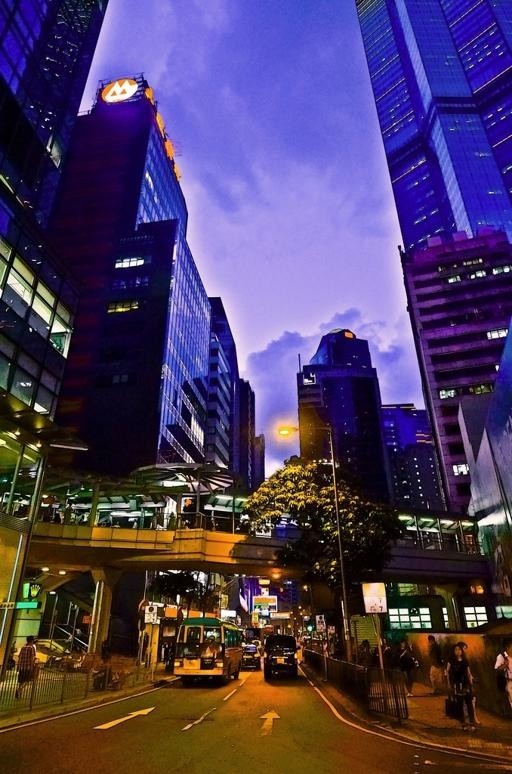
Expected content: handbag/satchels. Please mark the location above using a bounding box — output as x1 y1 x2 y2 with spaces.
445 699 458 720
494 653 508 690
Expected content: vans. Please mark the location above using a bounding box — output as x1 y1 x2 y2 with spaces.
264 636 298 681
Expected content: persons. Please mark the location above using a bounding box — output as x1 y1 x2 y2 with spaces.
0 494 113 528
376 633 420 698
156 642 175 663
16 635 36 699
101 640 112 663
67 630 77 643
428 635 481 734
494 636 512 708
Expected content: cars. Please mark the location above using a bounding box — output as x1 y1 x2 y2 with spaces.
240 645 261 672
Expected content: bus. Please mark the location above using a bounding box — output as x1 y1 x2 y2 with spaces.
173 617 241 683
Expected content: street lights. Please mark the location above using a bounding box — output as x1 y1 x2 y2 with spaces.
29 437 90 522
277 424 353 662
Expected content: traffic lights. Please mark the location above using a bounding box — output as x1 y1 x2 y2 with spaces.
306 621 314 632
